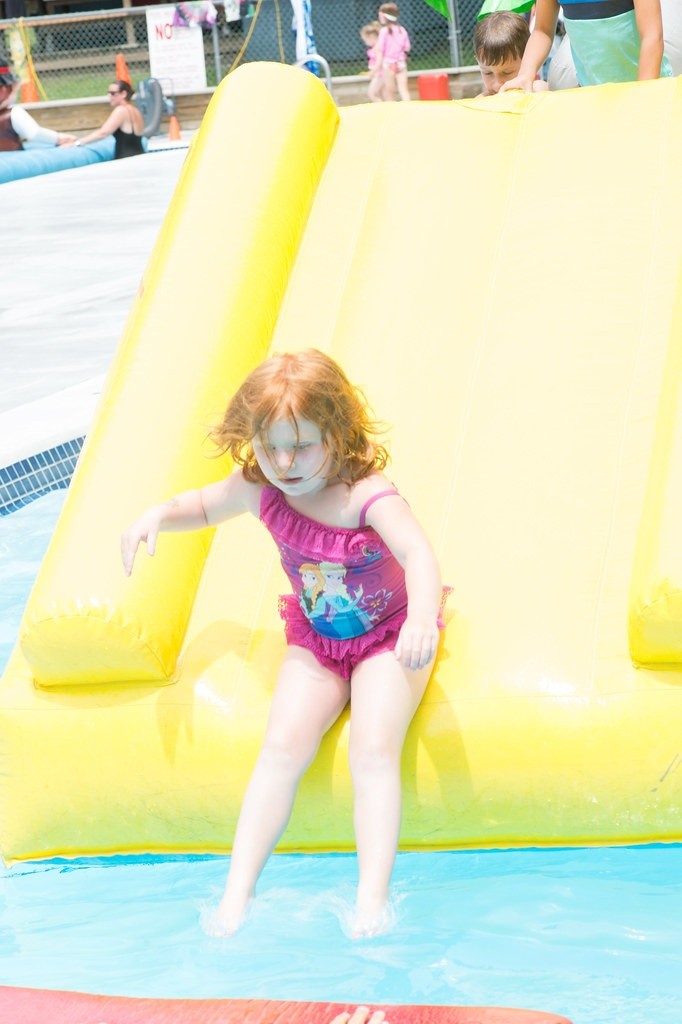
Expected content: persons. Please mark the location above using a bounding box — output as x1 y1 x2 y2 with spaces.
529 2 580 91
0 73 76 151
360 4 410 102
60 80 144 159
474 9 548 98
500 0 673 94
120 351 443 935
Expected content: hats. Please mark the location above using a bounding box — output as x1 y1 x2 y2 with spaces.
0 55 20 87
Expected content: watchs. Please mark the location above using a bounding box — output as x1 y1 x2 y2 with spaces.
74 140 80 146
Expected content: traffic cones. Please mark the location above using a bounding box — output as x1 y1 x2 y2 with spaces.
19 59 41 104
115 52 132 90
168 116 181 142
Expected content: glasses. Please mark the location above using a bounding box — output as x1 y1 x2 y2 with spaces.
107 90 119 96
